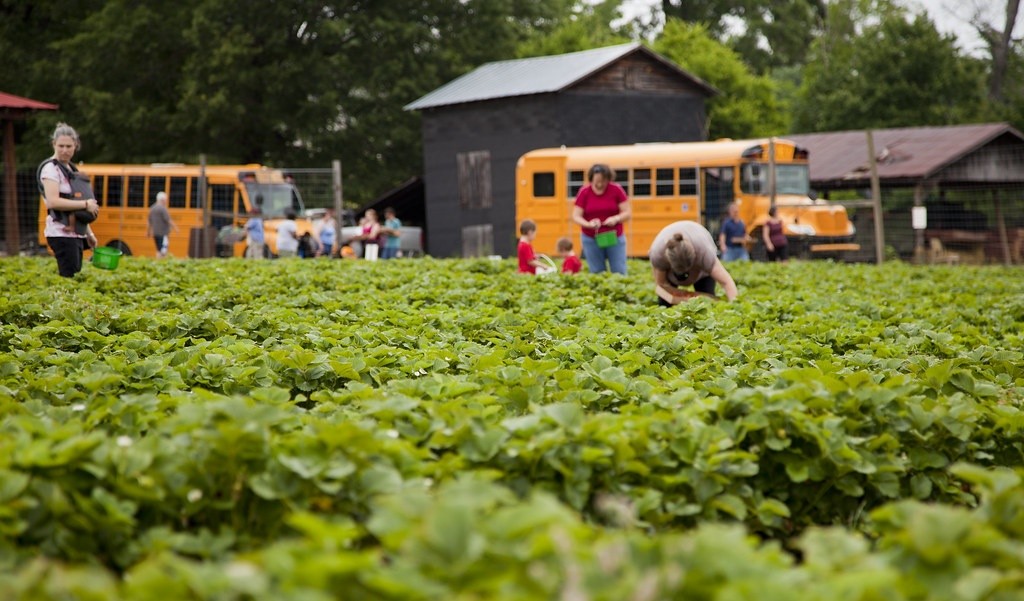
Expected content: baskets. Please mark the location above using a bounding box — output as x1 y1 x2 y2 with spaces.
595 223 617 247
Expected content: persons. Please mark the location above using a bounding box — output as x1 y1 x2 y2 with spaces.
558 239 582 273
649 221 737 308
147 191 177 258
763 206 787 260
353 209 380 258
315 212 334 255
380 208 402 259
564 164 630 268
719 205 749 260
36 123 99 277
240 210 264 259
517 220 548 274
275 213 304 257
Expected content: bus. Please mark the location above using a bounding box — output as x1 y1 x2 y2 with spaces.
39 162 323 258
515 138 861 261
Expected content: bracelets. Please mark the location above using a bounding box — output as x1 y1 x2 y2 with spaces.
88 234 94 237
86 201 88 208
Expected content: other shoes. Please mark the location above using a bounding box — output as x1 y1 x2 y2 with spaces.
296 230 318 257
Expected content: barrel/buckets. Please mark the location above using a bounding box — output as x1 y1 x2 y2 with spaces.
92 246 123 270
596 224 617 248
